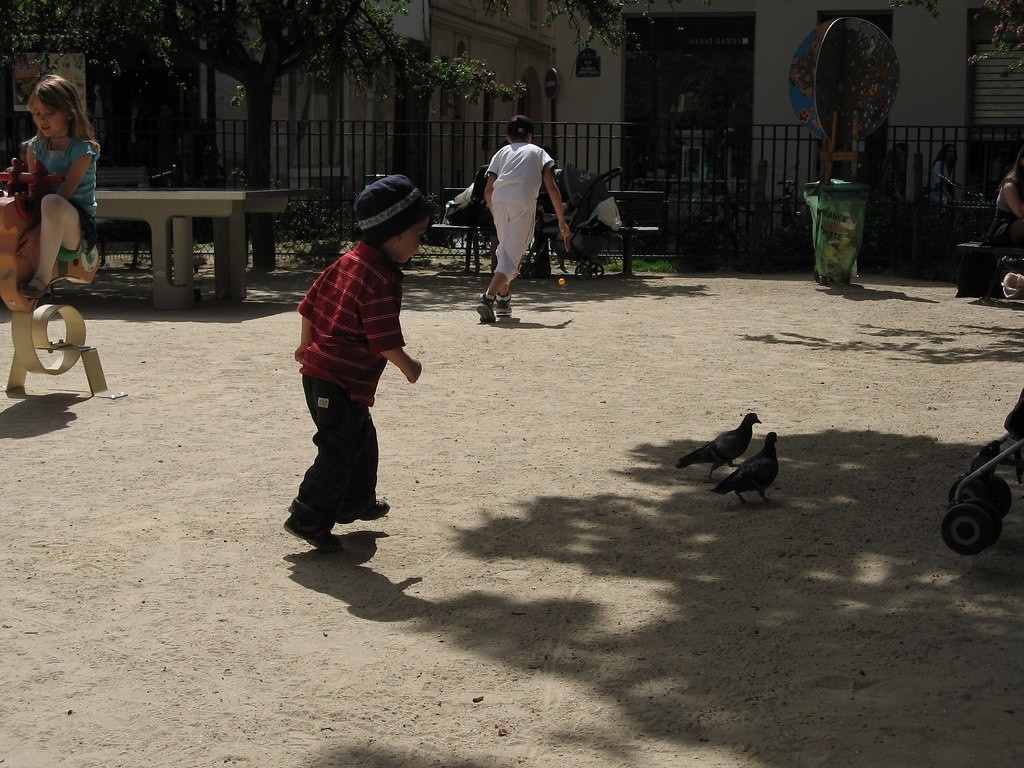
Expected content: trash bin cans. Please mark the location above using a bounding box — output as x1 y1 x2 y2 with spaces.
803 178 872 286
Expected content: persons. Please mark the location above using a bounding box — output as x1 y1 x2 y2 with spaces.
11 73 97 300
283 175 423 553
929 144 959 205
476 115 570 323
883 142 910 199
988 143 1024 248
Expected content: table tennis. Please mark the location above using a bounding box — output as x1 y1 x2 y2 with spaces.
558 278 565 286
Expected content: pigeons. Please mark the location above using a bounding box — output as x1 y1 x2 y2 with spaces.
675 413 762 482
710 431 780 508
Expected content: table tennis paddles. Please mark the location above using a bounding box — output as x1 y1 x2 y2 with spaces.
561 226 571 252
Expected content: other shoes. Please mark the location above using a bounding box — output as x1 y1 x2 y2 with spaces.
21 275 53 298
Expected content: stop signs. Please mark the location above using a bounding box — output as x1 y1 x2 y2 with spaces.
544 69 558 99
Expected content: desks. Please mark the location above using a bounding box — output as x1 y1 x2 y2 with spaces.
93 186 324 311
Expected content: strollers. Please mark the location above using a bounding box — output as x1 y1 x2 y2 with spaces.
941 384 1024 556
517 163 624 282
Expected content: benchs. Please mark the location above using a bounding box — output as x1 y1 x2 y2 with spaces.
98 167 154 272
956 203 1024 303
432 190 666 279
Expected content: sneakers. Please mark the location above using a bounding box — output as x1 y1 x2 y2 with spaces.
281 512 344 553
494 291 515 318
340 499 390 524
475 292 498 323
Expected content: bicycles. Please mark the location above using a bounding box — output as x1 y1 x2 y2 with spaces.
681 177 800 271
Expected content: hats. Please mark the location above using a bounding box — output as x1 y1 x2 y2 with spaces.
507 114 535 138
353 173 438 246
